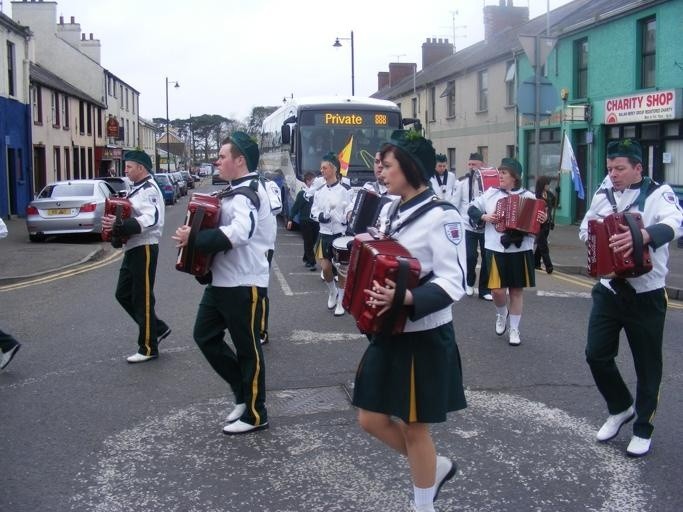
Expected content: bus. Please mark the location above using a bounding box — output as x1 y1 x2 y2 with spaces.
259 97 422 231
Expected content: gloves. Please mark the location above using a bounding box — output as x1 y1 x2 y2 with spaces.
321 210 331 220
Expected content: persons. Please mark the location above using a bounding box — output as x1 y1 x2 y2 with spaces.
532 174 558 275
430 153 458 201
579 141 683 456
468 157 548 347
344 149 398 233
0 217 23 372
309 153 354 317
303 155 353 282
258 168 278 345
353 131 465 512
102 151 171 363
287 172 320 271
272 169 289 227
169 132 270 435
451 153 494 301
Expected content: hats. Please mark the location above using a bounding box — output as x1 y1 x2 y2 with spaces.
435 153 447 162
384 127 436 183
500 156 523 176
468 151 484 162
122 149 153 171
321 151 339 168
228 128 260 171
605 137 643 164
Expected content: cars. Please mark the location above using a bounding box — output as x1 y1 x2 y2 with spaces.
153 173 180 205
211 170 230 185
100 177 136 194
198 167 207 177
178 171 196 189
166 171 187 196
191 172 201 181
25 179 126 240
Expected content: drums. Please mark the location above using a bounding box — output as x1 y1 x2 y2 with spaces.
470 166 500 229
330 234 356 266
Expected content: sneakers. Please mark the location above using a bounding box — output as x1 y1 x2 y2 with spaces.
320 269 324 279
625 435 652 458
303 259 308 266
547 266 553 276
482 293 493 301
535 265 541 269
1 342 21 368
433 455 457 503
157 327 171 345
326 287 340 310
126 351 160 366
334 298 346 316
223 402 248 423
223 418 270 436
333 274 340 282
257 330 268 344
595 405 636 442
509 329 521 346
310 263 316 271
495 309 510 336
465 284 474 296
